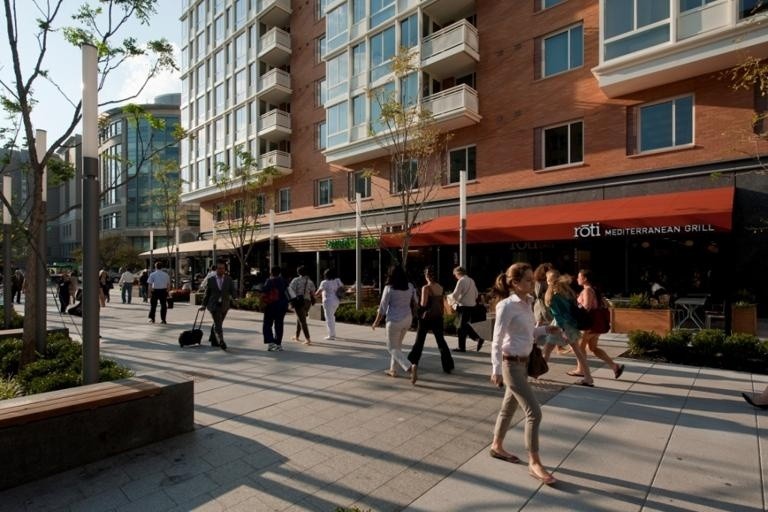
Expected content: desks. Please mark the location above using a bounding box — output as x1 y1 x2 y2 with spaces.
673 292 711 331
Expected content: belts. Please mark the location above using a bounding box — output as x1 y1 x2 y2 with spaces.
503 356 527 361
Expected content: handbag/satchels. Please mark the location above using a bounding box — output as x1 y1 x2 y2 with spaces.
410 291 419 329
469 304 486 324
336 279 345 299
528 343 549 379
290 295 305 308
571 308 594 330
590 306 610 334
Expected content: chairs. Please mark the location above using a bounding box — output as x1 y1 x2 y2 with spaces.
704 300 726 329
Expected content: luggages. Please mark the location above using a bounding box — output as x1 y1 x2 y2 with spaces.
179 307 205 347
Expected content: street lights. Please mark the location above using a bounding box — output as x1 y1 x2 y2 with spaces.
213 226 217 269
150 230 153 274
36 129 46 357
269 209 275 276
2 173 12 331
459 169 467 275
81 43 100 384
175 225 179 289
356 192 361 311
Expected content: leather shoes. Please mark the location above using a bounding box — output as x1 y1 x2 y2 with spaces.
452 348 466 352
490 447 520 465
476 338 484 351
528 463 557 485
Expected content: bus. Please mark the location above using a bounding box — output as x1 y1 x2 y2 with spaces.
46 257 80 282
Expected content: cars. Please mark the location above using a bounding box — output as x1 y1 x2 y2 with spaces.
77 264 175 284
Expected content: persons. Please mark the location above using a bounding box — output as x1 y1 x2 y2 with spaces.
198 263 239 350
288 264 315 344
315 267 345 340
373 261 419 384
447 264 485 353
741 384 767 411
408 264 455 375
475 263 626 389
10 259 176 324
489 262 557 484
261 266 290 352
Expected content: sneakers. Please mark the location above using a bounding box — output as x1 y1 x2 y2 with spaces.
410 364 417 384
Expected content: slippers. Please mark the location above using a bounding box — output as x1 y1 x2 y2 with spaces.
615 364 624 378
567 371 584 377
574 380 594 386
